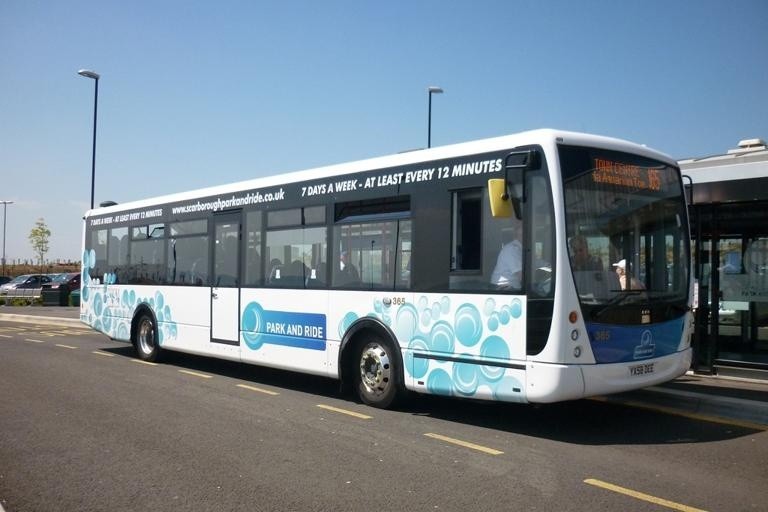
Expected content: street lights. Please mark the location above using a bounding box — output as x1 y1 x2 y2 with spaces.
427 86 444 148
78 69 100 210
0 200 13 276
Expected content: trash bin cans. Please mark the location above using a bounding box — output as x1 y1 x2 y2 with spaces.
42 283 69 305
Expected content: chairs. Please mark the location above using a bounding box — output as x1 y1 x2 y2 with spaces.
106 231 381 288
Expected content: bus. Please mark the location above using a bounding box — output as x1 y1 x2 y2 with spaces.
80 128 694 410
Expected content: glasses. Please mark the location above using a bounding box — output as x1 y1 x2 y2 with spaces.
573 247 585 253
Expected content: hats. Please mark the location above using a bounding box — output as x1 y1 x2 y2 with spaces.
612 259 632 272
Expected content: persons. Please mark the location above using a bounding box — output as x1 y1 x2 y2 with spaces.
489 220 537 290
221 235 237 275
340 251 359 280
612 259 647 291
569 236 602 274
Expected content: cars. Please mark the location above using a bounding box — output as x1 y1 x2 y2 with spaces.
0 272 80 296
